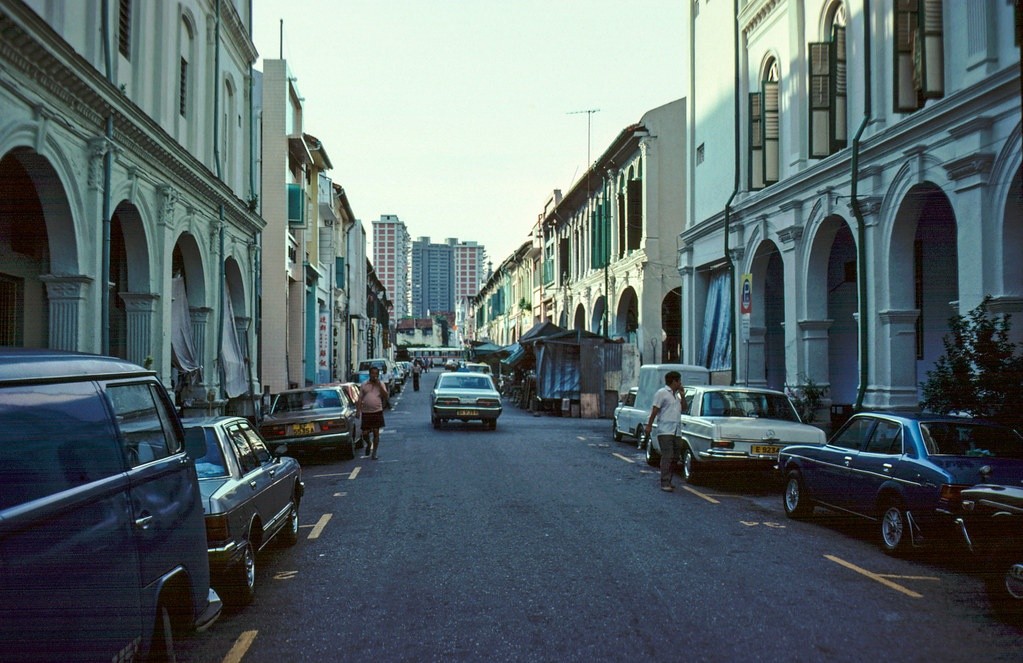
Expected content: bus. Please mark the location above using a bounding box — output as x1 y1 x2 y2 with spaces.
405 347 472 369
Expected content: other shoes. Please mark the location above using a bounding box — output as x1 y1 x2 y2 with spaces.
366 442 372 455
372 453 378 459
661 483 676 491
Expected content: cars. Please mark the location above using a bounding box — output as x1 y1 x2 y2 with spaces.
949 481 1023 626
430 356 495 378
771 407 1023 563
256 358 414 462
116 416 305 602
646 384 828 486
430 371 502 430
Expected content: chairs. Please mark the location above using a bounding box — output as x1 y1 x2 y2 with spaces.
477 378 488 388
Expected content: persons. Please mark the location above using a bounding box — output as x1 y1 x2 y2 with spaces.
356 367 389 461
412 361 420 392
450 359 458 366
408 357 429 377
645 371 688 491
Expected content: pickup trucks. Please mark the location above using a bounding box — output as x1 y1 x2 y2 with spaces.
614 386 654 453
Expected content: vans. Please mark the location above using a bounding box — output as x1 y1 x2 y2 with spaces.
0 349 224 663
